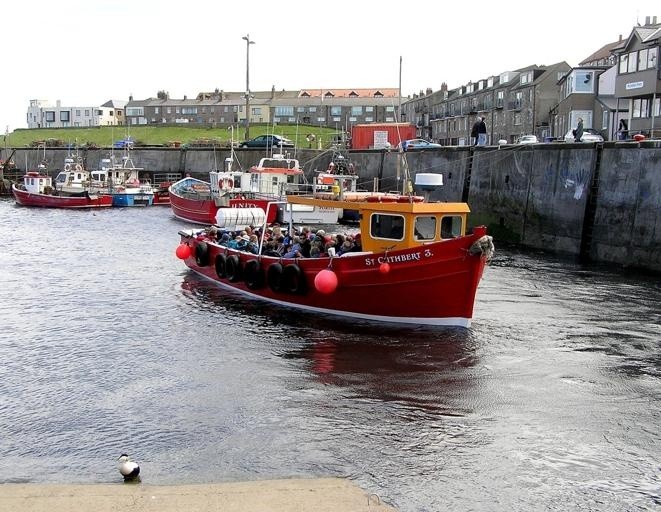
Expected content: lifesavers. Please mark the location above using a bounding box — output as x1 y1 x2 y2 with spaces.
75 164 82 172
64 163 71 171
306 132 315 143
26 171 38 176
367 195 423 203
218 176 233 191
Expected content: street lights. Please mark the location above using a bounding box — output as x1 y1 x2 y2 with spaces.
242 32 256 140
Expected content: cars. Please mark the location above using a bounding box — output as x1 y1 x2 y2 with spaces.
564 128 605 143
400 138 442 149
515 135 539 144
239 134 295 148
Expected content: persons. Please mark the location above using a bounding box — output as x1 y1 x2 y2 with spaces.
573 117 585 141
469 116 487 146
617 119 628 141
196 224 361 258
389 217 403 238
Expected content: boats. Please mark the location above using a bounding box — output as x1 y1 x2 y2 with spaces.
244 149 424 226
54 135 156 208
176 173 496 331
166 157 278 227
12 154 115 209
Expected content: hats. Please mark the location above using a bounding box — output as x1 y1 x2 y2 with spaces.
313 230 355 251
